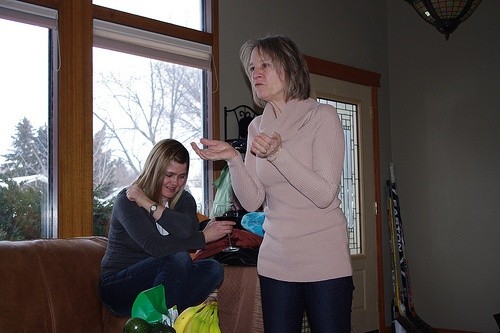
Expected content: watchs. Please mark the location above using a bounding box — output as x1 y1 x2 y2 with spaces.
150 203 159 216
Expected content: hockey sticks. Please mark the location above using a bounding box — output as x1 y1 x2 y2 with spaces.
384 162 435 333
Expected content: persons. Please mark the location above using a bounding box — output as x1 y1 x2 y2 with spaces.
190 35 356 333
99 138 236 318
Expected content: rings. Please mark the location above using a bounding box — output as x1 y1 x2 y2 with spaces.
264 149 267 154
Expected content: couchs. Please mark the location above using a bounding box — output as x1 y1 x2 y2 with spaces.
0 236 262 333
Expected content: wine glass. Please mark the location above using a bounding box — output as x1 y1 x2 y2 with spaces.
213 201 241 252
